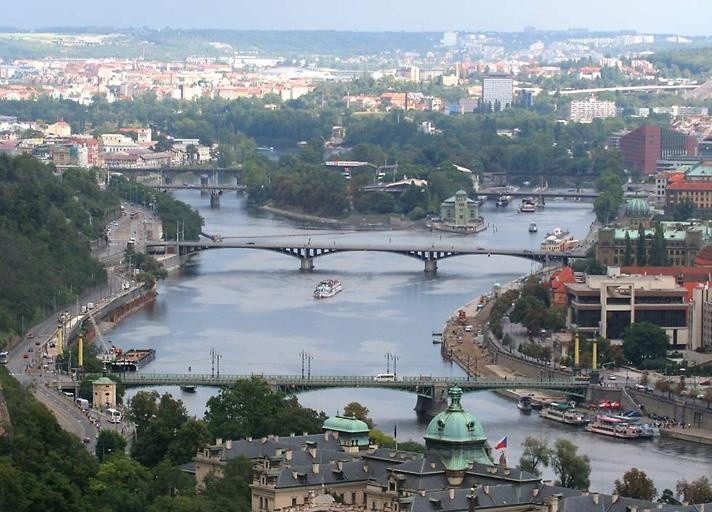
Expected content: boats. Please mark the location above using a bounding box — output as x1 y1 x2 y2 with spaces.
495 195 508 207
538 403 591 424
584 408 660 438
529 221 537 232
517 396 533 411
311 278 343 298
519 197 535 212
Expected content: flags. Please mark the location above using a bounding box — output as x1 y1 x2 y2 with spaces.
494 436 507 450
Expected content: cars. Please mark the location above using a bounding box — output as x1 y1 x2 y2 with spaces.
582 399 668 420
607 374 616 380
634 383 654 393
456 325 484 344
23 331 56 373
698 379 710 385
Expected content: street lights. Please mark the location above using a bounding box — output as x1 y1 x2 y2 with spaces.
383 350 399 379
298 349 314 381
209 346 222 379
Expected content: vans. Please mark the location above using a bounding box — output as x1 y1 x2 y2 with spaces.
372 374 395 382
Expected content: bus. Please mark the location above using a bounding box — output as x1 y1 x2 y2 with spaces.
0 351 10 364
63 391 75 401
75 398 90 409
106 409 122 423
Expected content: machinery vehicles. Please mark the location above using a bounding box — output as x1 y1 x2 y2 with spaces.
87 308 124 362
